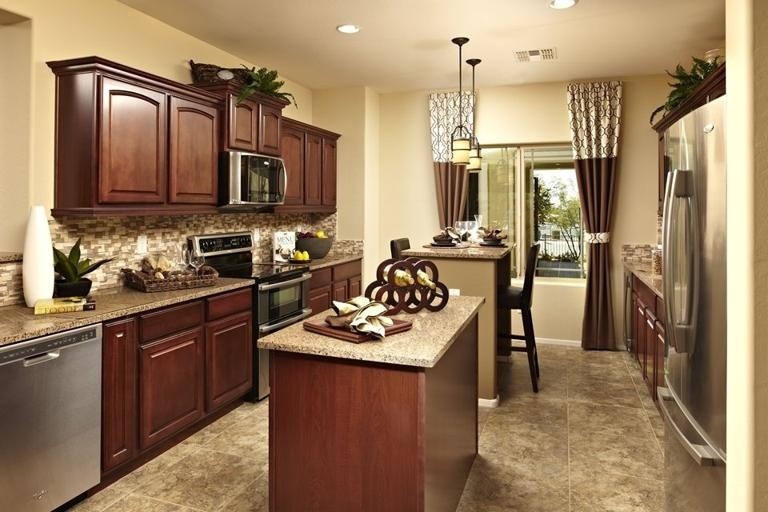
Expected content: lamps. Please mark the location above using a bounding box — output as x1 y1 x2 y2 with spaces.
462 57 482 171
451 36 473 165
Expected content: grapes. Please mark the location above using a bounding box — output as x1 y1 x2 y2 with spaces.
297 231 314 239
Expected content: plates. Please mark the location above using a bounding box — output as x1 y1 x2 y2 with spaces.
429 242 456 247
480 242 505 247
287 259 312 265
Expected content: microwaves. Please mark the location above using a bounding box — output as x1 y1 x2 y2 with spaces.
217 147 289 213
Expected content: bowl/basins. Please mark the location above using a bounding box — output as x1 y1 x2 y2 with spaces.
431 238 452 244
483 239 501 245
298 236 333 260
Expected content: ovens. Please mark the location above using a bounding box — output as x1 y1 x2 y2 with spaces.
245 270 313 404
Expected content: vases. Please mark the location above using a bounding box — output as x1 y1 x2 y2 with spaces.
20 205 59 308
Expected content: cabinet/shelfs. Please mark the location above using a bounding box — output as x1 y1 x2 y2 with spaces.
45 55 223 216
307 258 361 316
270 116 341 212
188 82 289 155
99 285 255 487
651 122 666 213
630 273 667 420
666 64 725 127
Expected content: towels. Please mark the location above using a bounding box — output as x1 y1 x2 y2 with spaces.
324 296 393 340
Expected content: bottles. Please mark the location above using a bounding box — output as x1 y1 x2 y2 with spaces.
652 243 662 276
383 265 415 287
408 268 437 291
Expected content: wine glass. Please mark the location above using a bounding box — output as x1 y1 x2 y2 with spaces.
162 243 205 278
456 215 484 243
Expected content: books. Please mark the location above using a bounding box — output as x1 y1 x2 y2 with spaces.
34 295 96 315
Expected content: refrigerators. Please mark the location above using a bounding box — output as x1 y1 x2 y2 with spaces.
654 94 727 511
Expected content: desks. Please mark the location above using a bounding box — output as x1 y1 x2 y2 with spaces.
256 289 484 512
400 240 516 408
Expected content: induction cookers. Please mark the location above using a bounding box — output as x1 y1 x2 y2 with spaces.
205 253 309 282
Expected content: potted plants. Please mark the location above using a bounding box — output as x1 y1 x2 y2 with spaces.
54 236 115 297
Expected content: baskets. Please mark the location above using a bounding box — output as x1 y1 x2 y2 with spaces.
120 265 220 293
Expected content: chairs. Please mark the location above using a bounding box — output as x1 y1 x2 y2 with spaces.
495 244 540 391
390 236 411 261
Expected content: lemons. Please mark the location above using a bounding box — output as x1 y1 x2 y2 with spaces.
317 231 324 237
295 250 309 261
